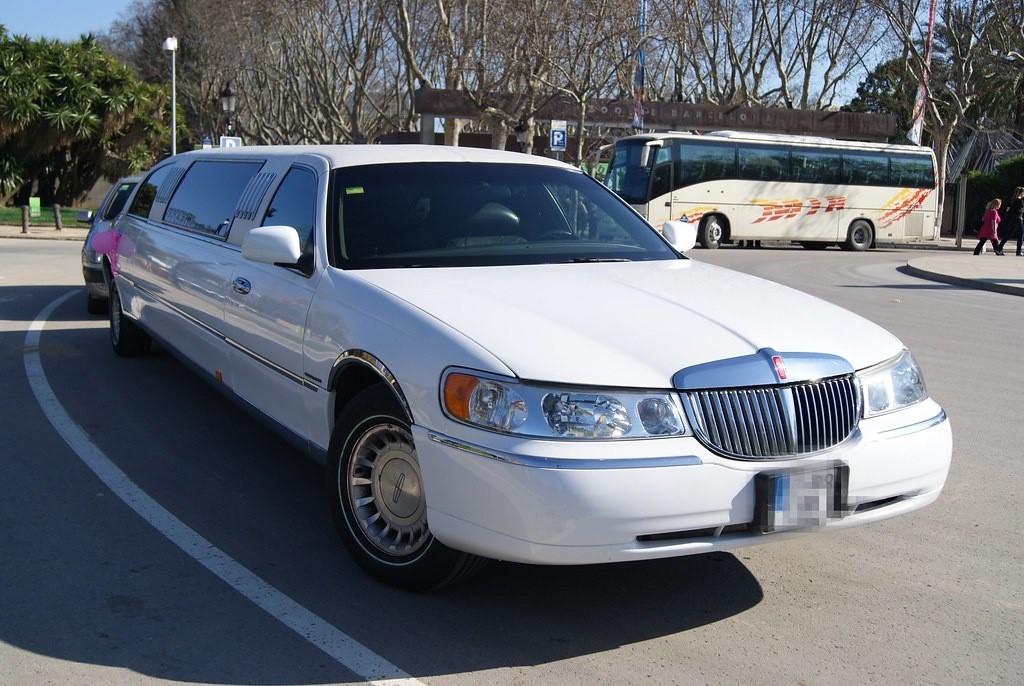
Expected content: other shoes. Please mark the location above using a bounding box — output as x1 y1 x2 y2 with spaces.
998 248 1004 255
1016 251 1024 256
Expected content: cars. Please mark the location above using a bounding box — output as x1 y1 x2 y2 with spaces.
78 143 953 580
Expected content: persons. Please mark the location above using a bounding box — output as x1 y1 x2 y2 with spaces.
995 186 1024 256
972 199 1003 256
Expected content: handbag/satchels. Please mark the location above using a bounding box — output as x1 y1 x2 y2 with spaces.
980 243 986 255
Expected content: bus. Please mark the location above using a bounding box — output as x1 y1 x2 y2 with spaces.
594 130 939 251
